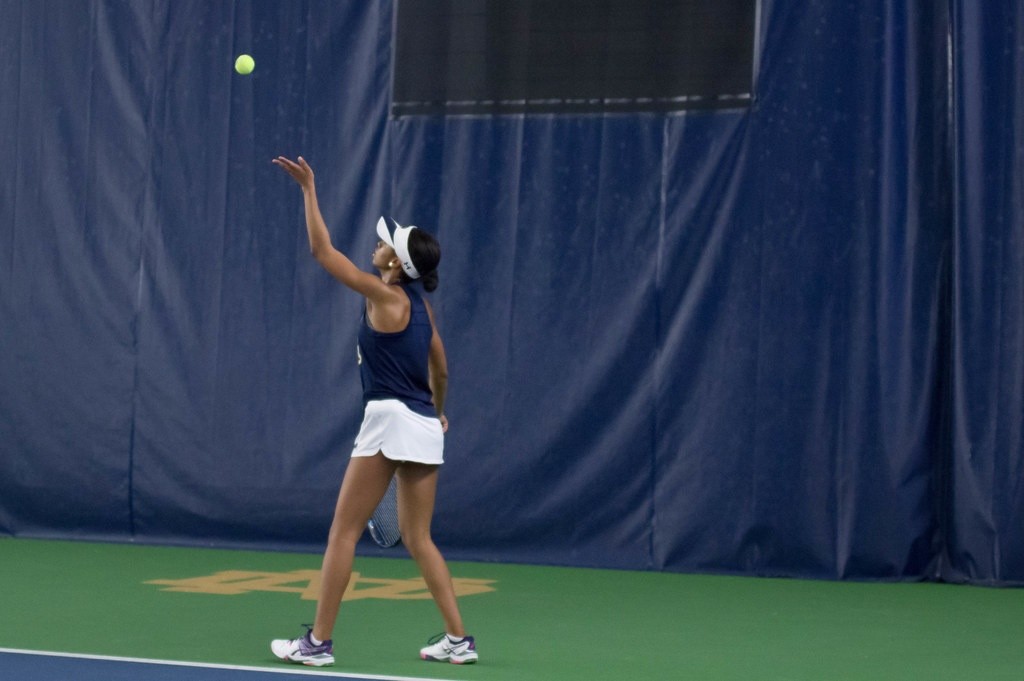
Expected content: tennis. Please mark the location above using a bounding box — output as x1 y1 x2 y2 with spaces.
235 54 255 75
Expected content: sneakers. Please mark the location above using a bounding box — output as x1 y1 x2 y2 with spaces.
270 624 335 667
419 631 479 664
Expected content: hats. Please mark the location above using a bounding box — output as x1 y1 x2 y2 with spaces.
376 216 421 280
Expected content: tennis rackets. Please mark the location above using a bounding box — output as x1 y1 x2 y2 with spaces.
368 476 401 548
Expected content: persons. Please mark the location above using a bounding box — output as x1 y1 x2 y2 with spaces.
270 154 478 665
